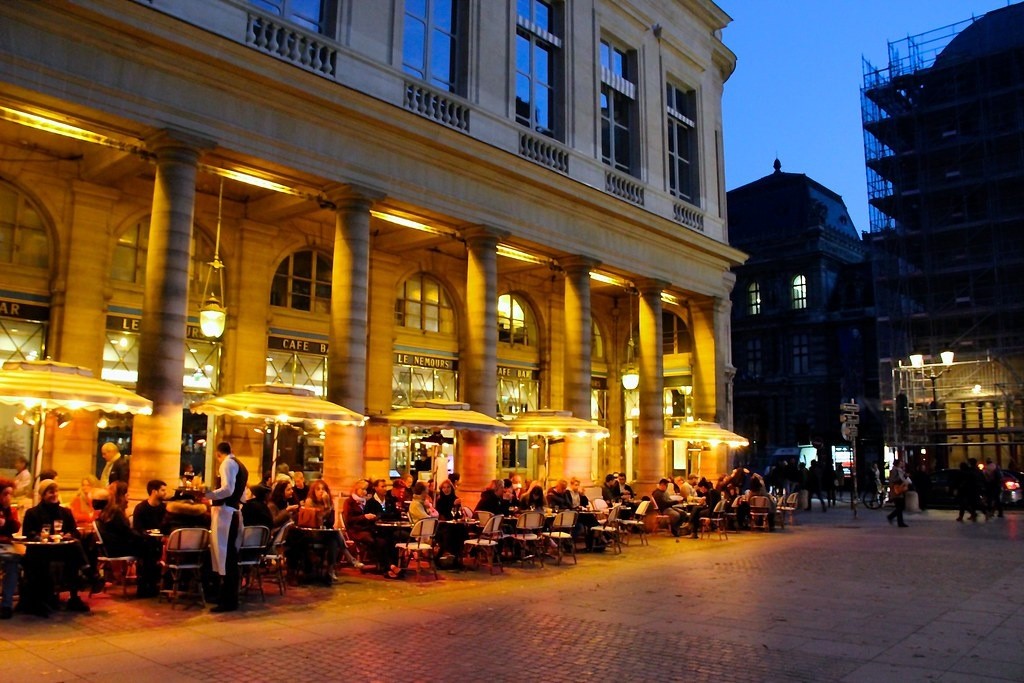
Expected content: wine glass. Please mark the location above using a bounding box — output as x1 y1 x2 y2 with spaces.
193 475 201 489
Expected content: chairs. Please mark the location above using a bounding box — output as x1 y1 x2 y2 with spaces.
543 511 578 566
476 510 513 562
396 518 439 583
91 520 135 598
250 540 288 596
726 496 742 534
591 497 672 551
157 528 209 609
511 512 546 569
237 526 269 606
701 498 728 541
750 492 800 532
465 514 505 574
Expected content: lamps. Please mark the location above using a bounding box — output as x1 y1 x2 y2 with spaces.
200 176 227 338
621 288 638 390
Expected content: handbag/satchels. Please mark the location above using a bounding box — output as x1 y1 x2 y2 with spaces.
894 481 908 494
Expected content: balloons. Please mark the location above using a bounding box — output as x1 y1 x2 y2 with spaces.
664 418 749 481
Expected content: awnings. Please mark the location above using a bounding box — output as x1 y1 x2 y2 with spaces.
772 447 800 456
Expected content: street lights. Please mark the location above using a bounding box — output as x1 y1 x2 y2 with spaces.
898 351 954 467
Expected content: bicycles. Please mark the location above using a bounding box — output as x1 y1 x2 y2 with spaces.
863 486 884 510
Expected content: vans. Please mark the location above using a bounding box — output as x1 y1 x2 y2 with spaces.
912 469 1023 509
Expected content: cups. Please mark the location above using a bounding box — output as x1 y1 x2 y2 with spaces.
53 519 63 534
555 506 559 513
185 482 192 491
41 524 51 542
547 508 552 515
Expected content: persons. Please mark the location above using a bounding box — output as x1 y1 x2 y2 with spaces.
0 440 1024 618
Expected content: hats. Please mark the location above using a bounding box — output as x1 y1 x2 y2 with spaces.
88 489 110 500
38 479 58 496
606 474 618 482
690 473 699 480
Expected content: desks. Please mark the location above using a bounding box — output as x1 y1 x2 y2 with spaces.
440 519 480 528
292 526 344 585
374 521 412 566
674 502 700 509
580 510 605 518
13 533 82 622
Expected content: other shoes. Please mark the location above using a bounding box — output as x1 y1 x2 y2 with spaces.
66 597 90 612
679 525 689 531
391 566 404 576
0 600 13 620
209 600 238 612
687 533 697 538
136 586 160 599
385 570 400 579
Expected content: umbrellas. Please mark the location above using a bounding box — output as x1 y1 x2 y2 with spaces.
367 398 509 509
191 385 366 487
0 356 152 509
494 407 610 495
